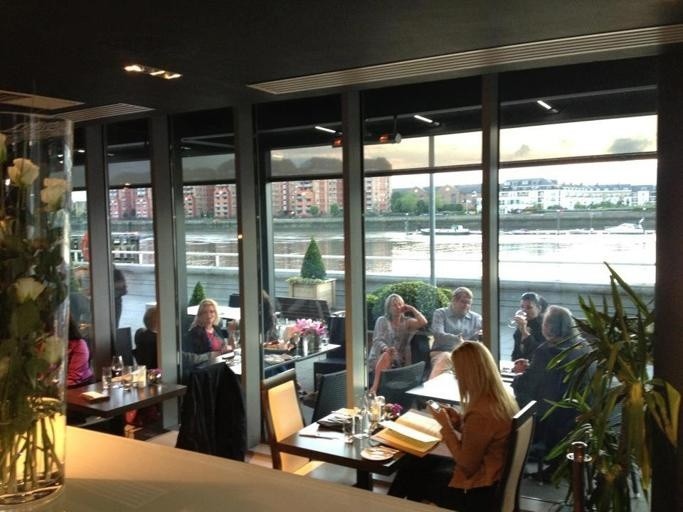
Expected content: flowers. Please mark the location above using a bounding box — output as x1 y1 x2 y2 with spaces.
285 317 329 340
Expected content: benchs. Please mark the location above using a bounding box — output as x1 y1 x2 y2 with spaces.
229 294 345 330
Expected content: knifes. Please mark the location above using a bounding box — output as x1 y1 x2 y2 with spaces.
297 434 338 440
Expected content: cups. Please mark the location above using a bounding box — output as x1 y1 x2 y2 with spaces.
340 390 385 444
99 353 146 390
233 349 241 361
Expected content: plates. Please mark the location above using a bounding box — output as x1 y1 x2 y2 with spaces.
360 447 393 460
263 357 284 365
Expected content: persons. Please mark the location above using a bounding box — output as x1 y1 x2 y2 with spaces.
182 297 228 355
386 341 519 510
112 263 129 329
62 315 92 386
257 289 288 378
78 232 90 297
366 294 427 394
429 286 483 381
510 305 598 465
510 290 549 360
134 308 218 379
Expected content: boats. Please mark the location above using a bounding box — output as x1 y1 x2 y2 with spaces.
419 224 469 235
602 222 644 235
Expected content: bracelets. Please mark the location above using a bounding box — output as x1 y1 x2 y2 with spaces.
415 312 423 316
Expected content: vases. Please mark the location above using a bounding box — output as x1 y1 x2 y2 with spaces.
296 332 320 355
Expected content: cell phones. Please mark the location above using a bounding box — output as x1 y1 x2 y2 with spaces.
425 399 441 411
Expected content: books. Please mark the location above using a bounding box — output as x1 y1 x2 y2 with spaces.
372 408 446 457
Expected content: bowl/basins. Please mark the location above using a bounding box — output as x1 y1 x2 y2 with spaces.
264 342 294 354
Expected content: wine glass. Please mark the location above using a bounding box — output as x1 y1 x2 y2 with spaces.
507 310 525 329
232 330 239 348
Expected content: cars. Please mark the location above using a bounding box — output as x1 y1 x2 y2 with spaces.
363 209 536 218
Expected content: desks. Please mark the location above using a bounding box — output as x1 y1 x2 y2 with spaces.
217 332 340 392
64 372 188 438
403 368 519 409
275 409 407 493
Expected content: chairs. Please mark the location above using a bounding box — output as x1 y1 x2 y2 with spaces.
493 399 538 510
81 338 95 369
311 370 346 425
259 367 312 475
172 362 246 462
313 360 347 389
409 334 433 379
378 361 426 415
116 326 134 371
302 318 347 409
77 414 126 437
264 362 297 380
